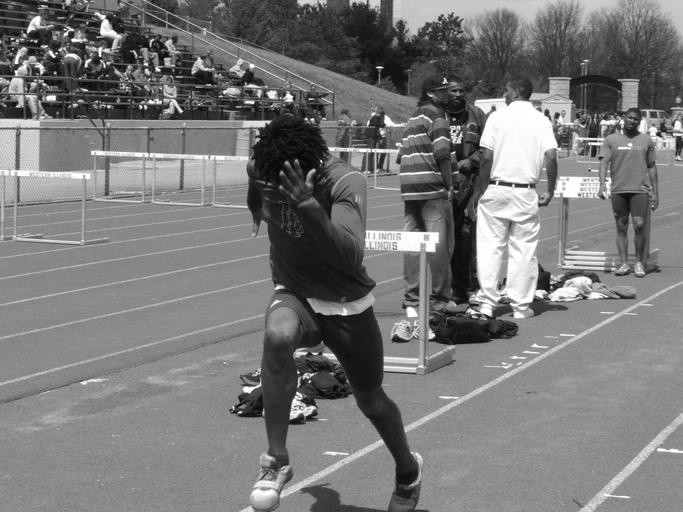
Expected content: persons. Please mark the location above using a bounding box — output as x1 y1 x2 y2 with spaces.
307 83 330 118
639 114 648 134
444 75 488 308
163 77 183 118
339 106 352 164
403 74 457 318
228 59 257 86
0 5 106 119
192 52 216 87
204 46 215 68
486 104 497 116
361 106 388 174
598 108 659 277
659 120 667 139
368 104 407 172
119 65 162 110
100 11 183 74
570 110 627 155
673 115 683 161
649 124 657 137
535 106 566 128
479 75 557 319
247 115 423 510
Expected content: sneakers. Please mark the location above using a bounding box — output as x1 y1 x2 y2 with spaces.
250 454 293 511
406 306 419 317
676 156 682 161
240 368 264 386
389 453 424 511
262 396 305 424
635 262 645 276
445 301 469 313
480 308 493 318
513 309 534 318
289 399 318 420
411 322 436 341
390 318 413 341
615 264 631 275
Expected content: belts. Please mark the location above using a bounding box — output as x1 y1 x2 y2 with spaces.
489 180 535 188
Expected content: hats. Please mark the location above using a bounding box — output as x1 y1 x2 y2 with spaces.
93 53 98 59
423 75 455 90
38 5 50 9
249 63 254 69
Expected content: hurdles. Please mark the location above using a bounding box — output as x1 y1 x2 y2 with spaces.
210 155 252 209
1 169 31 241
587 142 611 174
553 176 660 272
652 140 672 166
372 149 400 191
576 137 612 164
673 134 683 167
10 169 111 246
295 230 457 366
149 152 220 207
327 146 374 190
90 150 160 204
293 239 454 376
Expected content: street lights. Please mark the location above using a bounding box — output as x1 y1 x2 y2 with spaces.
375 66 384 86
580 63 584 110
583 59 590 112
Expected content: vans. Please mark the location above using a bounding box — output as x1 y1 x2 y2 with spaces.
640 110 672 128
